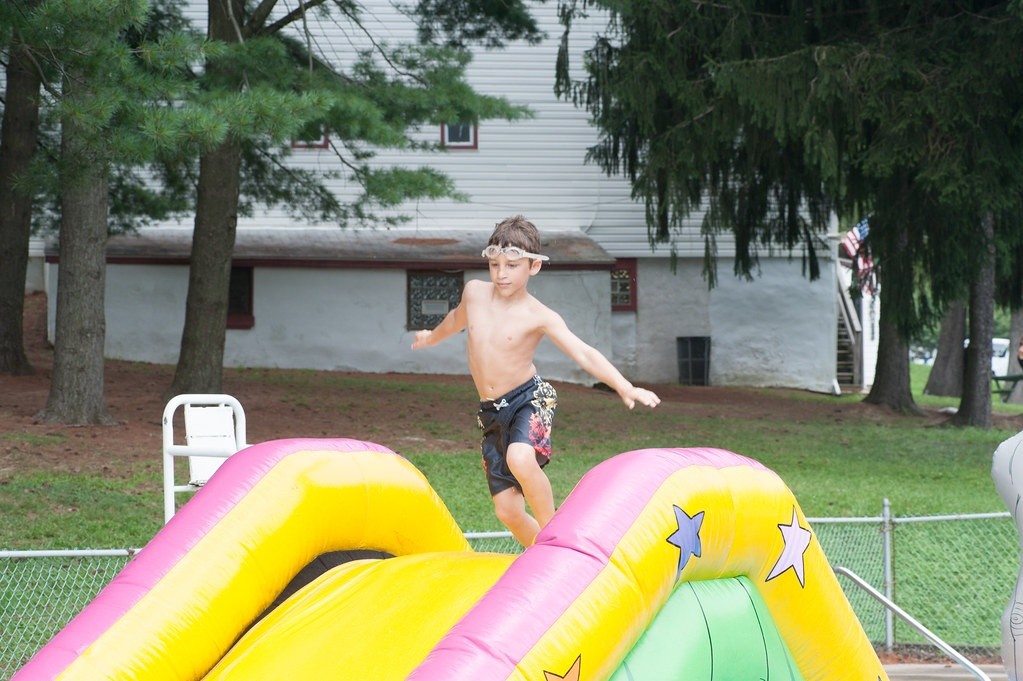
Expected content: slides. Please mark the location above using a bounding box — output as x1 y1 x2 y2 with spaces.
8 438 896 681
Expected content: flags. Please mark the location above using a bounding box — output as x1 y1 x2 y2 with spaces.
841 212 886 299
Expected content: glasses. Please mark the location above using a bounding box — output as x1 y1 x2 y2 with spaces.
481 245 549 261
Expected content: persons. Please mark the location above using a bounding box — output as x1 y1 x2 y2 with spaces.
410 213 662 557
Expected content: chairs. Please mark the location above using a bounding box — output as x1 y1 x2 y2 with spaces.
162 394 255 530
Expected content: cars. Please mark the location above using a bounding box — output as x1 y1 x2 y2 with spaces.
964 337 1010 377
